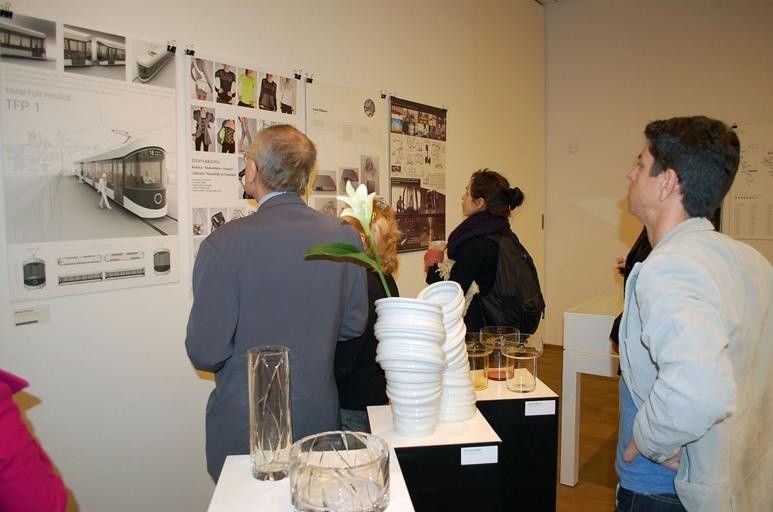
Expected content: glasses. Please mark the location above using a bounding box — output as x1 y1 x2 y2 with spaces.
242 151 258 173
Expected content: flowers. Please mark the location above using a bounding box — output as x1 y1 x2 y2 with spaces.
305 180 406 298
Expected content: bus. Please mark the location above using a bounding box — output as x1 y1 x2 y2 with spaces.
0 21 47 62
63 29 93 67
134 45 173 84
20 258 46 292
150 246 171 275
94 37 126 67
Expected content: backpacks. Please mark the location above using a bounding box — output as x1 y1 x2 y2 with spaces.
472 230 545 343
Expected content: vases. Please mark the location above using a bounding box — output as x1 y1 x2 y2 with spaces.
371 283 474 433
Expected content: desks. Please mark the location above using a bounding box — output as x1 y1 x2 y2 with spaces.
210 367 557 511
558 290 631 484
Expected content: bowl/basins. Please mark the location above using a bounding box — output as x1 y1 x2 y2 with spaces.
371 279 479 436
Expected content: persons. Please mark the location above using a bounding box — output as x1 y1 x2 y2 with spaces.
236 68 255 105
214 65 235 105
142 170 153 181
396 195 403 213
408 194 415 211
336 204 398 431
237 117 251 152
610 203 723 377
190 58 212 101
185 125 368 486
425 171 523 367
614 114 773 512
192 107 212 151
258 74 276 111
218 119 234 151
97 173 111 209
279 78 296 114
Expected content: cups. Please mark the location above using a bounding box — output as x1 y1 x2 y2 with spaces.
288 430 391 511
246 344 291 482
463 325 545 393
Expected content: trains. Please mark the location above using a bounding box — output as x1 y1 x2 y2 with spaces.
71 126 170 221
